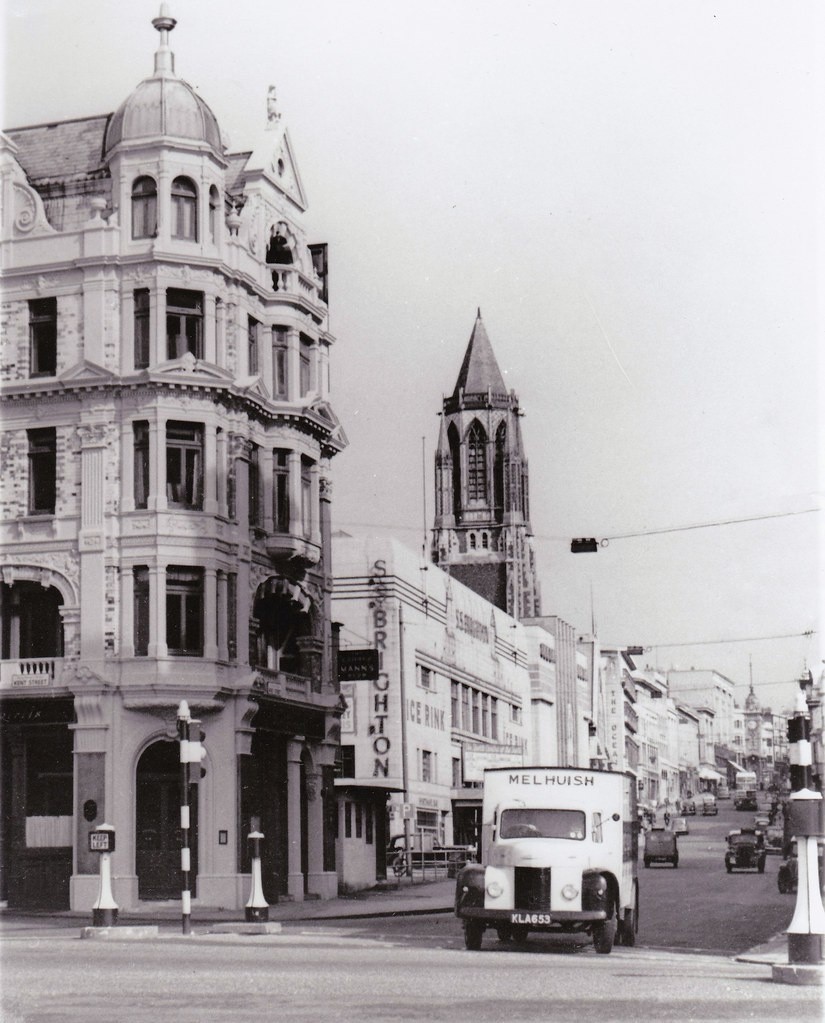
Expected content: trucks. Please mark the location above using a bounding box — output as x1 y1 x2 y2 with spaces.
449 762 641 953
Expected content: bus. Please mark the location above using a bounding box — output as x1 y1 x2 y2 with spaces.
736 772 757 790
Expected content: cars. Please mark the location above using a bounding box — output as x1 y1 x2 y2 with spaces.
776 837 824 893
755 810 787 853
387 832 474 868
644 829 679 868
703 796 718 814
717 787 731 799
680 799 694 815
734 790 758 811
726 829 766 873
